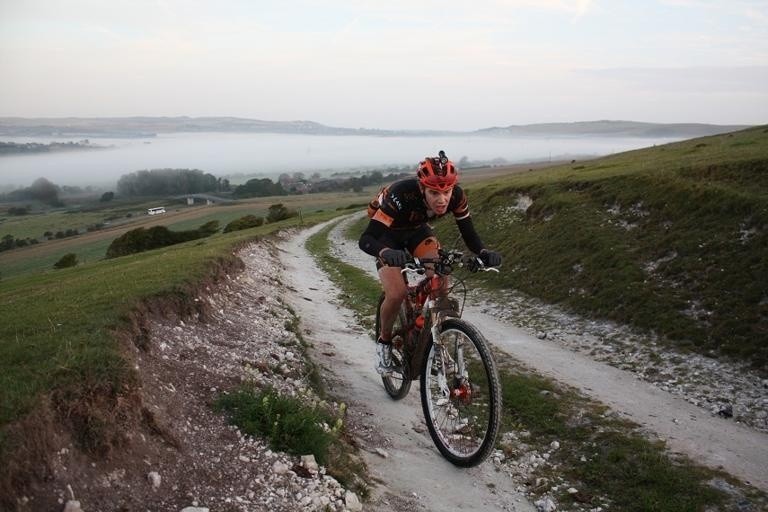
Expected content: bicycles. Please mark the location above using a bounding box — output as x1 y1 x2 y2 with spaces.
372 242 510 463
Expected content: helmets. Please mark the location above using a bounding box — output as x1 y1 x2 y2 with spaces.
416 157 458 192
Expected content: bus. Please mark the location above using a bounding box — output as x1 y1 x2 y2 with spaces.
147 207 166 214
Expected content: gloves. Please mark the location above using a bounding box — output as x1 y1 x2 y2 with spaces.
382 249 406 267
481 250 501 267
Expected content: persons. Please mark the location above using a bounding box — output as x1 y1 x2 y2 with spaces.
359 151 502 379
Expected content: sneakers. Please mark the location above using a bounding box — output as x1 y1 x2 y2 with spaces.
443 349 457 375
375 341 393 376
